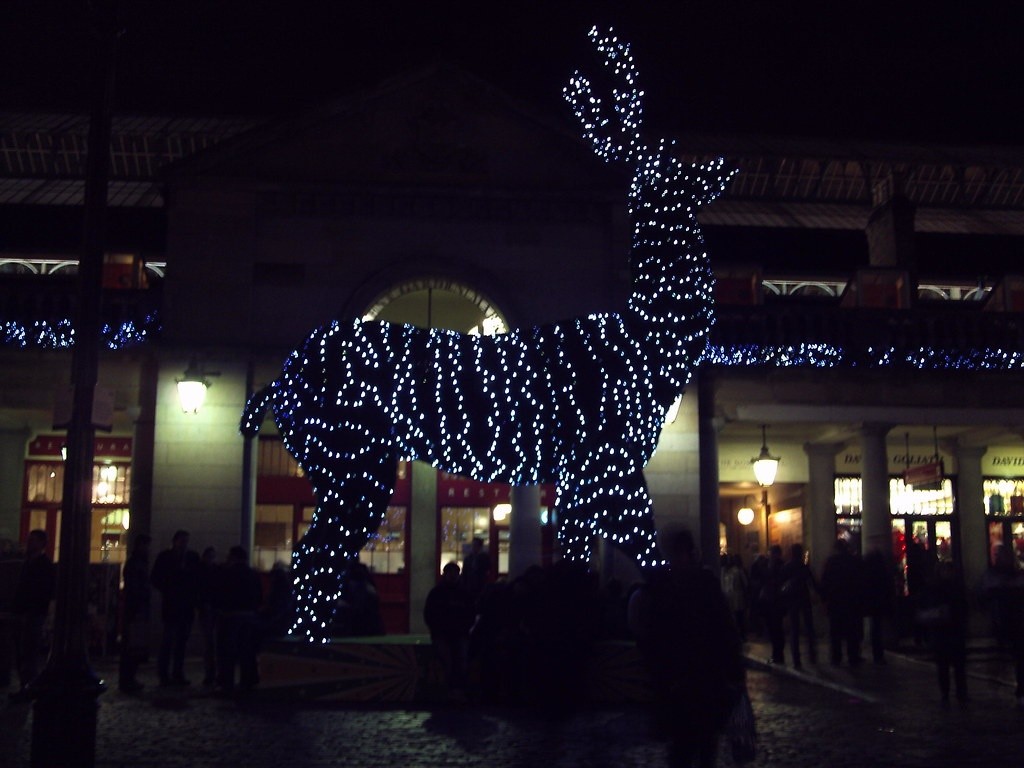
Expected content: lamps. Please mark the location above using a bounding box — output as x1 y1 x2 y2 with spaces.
737 496 755 525
174 358 213 414
752 423 779 487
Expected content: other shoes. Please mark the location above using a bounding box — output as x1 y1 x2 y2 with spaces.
939 689 951 708
202 671 217 685
118 674 145 691
173 673 192 685
954 687 969 700
159 670 178 687
7 685 33 701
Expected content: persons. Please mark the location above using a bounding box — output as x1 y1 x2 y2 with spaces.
422 536 487 702
719 539 1024 706
338 565 386 637
8 530 56 704
119 530 289 691
630 527 747 768
